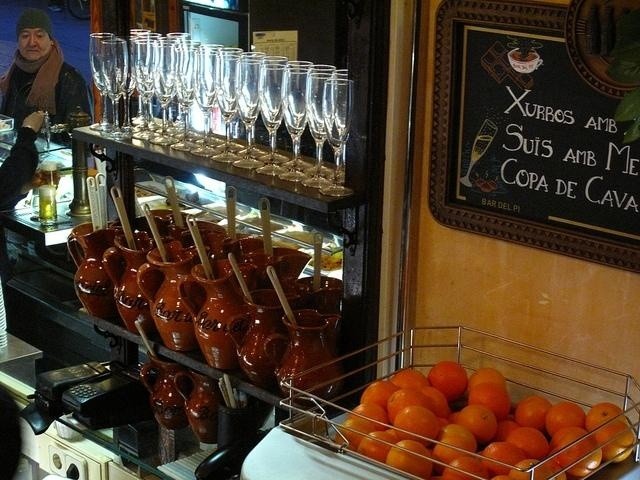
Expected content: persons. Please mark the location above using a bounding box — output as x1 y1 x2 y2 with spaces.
1 111 47 288
1 7 94 148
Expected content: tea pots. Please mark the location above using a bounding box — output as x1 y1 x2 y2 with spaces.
140 349 192 430
172 366 225 445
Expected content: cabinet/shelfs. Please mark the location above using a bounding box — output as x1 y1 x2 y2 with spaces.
0 122 380 480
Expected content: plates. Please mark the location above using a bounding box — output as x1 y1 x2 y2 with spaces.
0 277 8 349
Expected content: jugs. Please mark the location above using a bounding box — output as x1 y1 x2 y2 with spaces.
66 208 345 409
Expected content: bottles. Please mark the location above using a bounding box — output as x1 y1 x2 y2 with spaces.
64 103 92 140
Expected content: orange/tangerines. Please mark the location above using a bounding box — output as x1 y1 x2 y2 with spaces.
334 361 639 480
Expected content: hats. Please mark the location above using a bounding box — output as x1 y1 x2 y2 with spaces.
16 7 53 43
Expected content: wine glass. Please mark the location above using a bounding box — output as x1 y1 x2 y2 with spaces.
89 28 354 200
459 120 500 188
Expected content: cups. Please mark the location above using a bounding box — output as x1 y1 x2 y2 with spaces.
508 50 544 73
38 184 58 228
41 161 61 192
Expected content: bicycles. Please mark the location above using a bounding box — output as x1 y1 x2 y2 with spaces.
67 0 92 20
